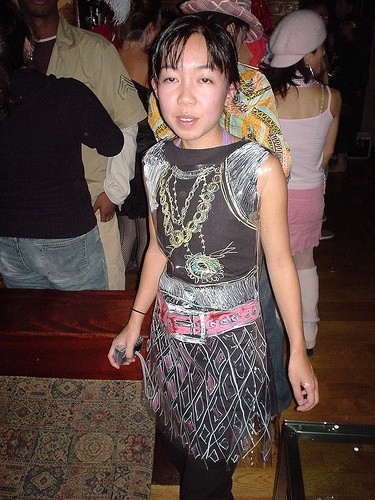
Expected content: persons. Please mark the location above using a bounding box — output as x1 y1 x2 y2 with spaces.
267 10 342 355
104 0 162 277
146 0 290 183
0 0 124 292
108 16 319 500
19 0 148 290
300 0 372 241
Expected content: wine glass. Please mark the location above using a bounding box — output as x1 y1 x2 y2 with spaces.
96 7 103 25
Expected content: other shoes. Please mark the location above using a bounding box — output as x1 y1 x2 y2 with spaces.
319 229 334 240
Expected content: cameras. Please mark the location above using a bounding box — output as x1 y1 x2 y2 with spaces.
114 335 145 366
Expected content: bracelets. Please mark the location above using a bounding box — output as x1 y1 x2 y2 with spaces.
132 308 146 315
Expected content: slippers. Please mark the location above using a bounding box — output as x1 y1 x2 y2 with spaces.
327 159 349 176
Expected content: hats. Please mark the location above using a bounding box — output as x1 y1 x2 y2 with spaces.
180 0 264 43
269 11 325 68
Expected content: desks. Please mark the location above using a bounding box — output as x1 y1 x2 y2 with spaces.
0 286 157 500
